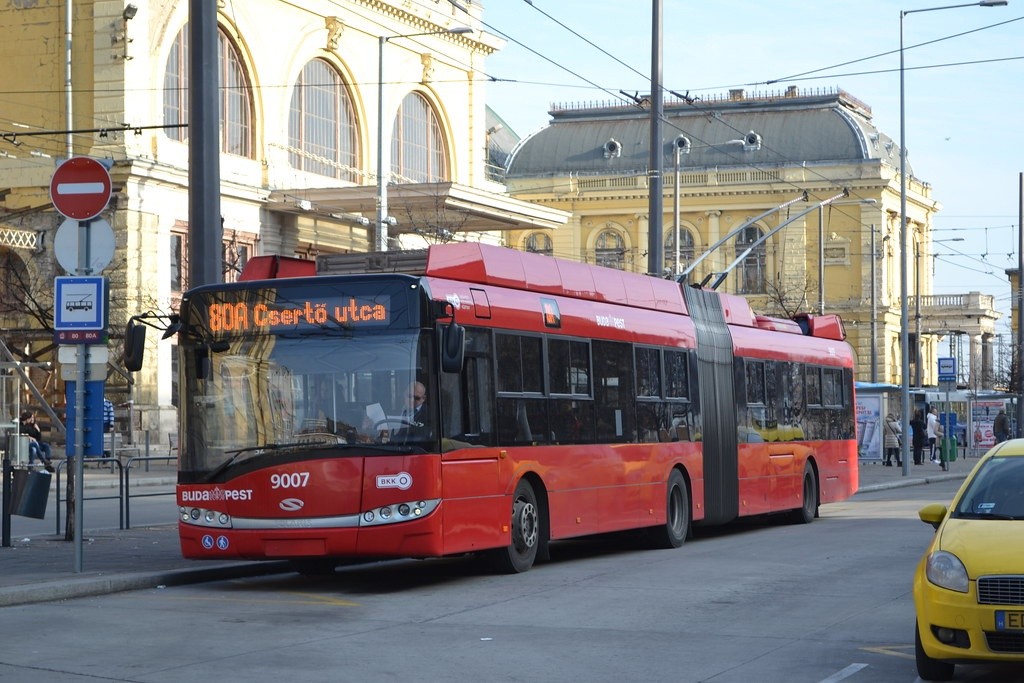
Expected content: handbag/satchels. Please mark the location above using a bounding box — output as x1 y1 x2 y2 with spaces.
898 438 903 446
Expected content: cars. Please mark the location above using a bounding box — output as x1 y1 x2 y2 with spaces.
912 436 1024 683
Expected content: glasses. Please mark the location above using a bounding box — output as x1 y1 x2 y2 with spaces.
407 394 422 401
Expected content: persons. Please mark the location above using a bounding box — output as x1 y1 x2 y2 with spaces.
883 413 902 466
101 396 114 463
993 409 1009 443
934 421 947 471
362 381 426 431
19 411 55 473
910 410 927 465
927 407 938 461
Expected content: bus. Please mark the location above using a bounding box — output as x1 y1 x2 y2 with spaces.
122 186 860 579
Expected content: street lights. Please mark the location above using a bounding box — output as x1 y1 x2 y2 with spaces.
818 199 876 315
674 137 745 276
897 0 1011 476
916 236 964 385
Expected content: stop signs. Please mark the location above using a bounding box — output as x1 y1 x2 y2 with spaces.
48 156 113 222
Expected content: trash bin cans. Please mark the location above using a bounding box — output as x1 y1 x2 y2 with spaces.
16 470 51 519
942 438 957 461
8 470 32 514
9 434 29 470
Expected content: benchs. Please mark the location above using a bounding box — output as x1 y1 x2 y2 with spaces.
104 433 140 468
167 433 179 465
899 436 931 464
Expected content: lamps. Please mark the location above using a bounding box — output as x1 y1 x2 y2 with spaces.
124 4 138 21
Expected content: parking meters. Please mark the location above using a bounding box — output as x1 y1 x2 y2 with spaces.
374 28 475 252
